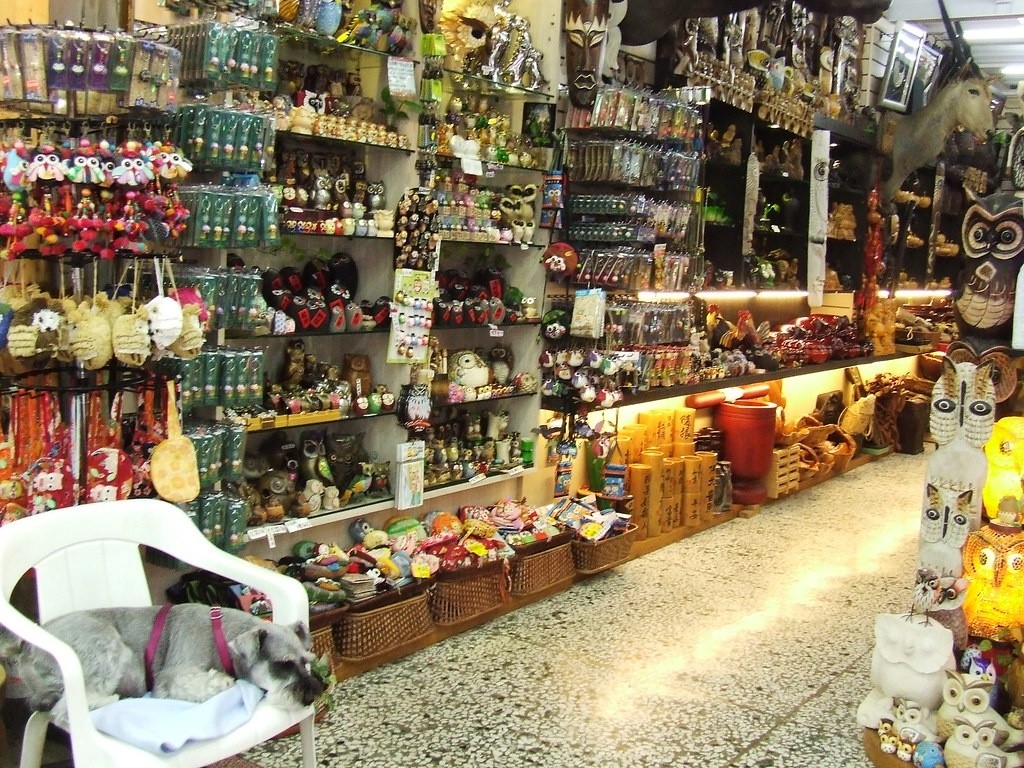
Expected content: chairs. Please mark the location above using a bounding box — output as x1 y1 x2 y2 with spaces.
0 479 324 768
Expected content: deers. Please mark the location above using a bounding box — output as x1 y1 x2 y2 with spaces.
875 56 1006 207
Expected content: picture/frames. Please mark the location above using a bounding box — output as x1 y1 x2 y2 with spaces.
915 45 944 86
875 19 929 113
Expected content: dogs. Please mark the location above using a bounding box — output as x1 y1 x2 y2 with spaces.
17 602 324 712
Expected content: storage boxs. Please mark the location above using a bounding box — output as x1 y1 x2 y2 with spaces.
766 445 803 500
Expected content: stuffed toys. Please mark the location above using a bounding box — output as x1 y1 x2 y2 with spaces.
498 182 624 458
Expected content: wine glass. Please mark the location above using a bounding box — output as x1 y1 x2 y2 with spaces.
710 399 778 505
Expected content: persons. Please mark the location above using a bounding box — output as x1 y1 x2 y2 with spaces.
564 0 608 108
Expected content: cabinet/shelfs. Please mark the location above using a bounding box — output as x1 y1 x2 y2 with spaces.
156 0 1010 681
